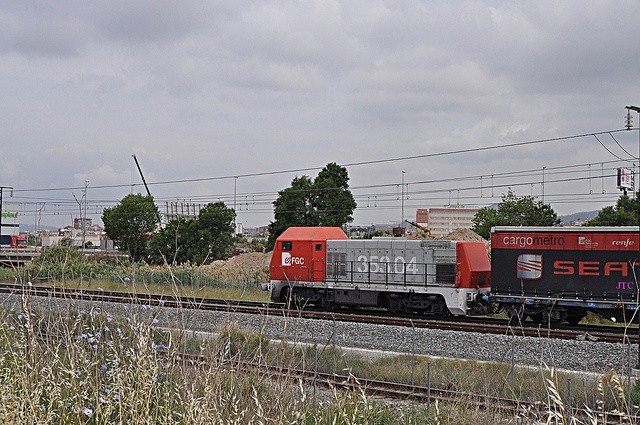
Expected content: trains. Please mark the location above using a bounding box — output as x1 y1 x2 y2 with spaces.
262 226 640 324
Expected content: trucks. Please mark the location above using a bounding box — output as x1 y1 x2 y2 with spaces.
1 222 30 249
